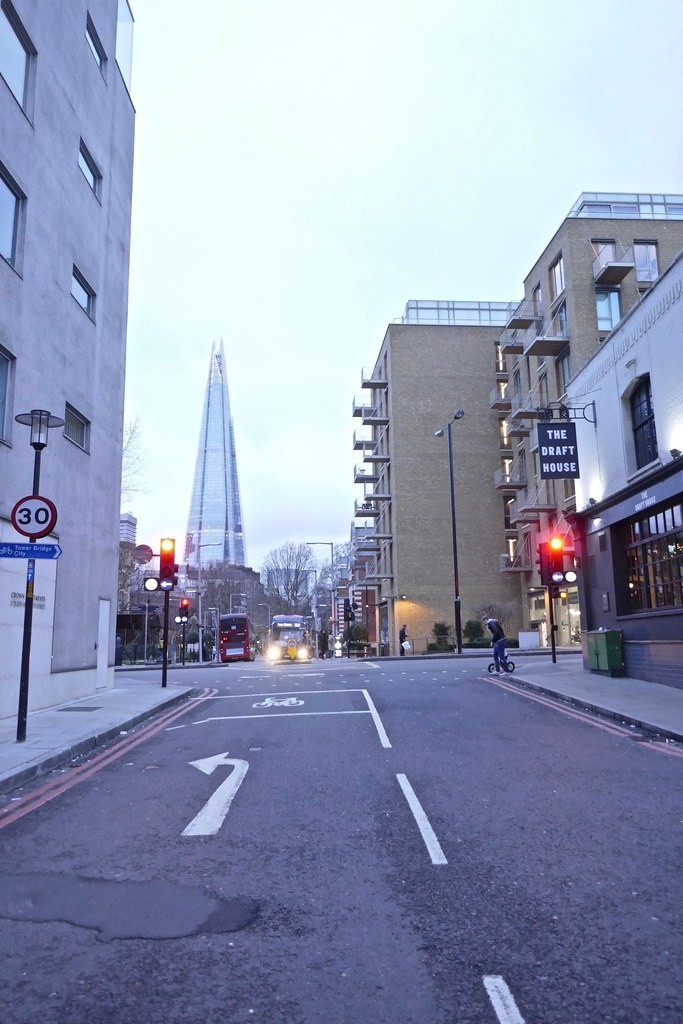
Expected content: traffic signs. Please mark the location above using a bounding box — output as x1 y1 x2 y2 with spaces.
0 542 64 560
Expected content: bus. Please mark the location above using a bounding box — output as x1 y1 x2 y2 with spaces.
269 614 311 658
218 613 257 662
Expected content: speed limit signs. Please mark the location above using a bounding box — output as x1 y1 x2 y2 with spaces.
11 495 58 539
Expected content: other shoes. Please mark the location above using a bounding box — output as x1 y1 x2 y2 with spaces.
489 670 501 676
499 671 511 676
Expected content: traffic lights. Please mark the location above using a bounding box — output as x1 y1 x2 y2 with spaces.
534 541 553 587
175 598 190 624
550 585 567 598
548 536 578 585
172 563 179 586
343 598 356 622
142 538 176 592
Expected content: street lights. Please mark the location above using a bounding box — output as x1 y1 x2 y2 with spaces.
140 601 149 665
257 603 271 644
12 409 68 743
300 568 319 660
207 606 220 661
229 594 247 614
305 541 336 658
197 542 223 662
434 409 467 654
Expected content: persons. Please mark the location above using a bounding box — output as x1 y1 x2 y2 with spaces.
481 615 512 677
317 630 327 660
188 649 199 663
398 625 409 656
325 631 337 659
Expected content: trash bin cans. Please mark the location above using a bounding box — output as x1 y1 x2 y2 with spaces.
587 628 626 678
116 633 124 665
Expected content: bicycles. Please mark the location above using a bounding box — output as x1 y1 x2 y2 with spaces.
488 653 516 674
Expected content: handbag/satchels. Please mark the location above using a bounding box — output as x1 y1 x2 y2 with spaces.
401 641 411 650
